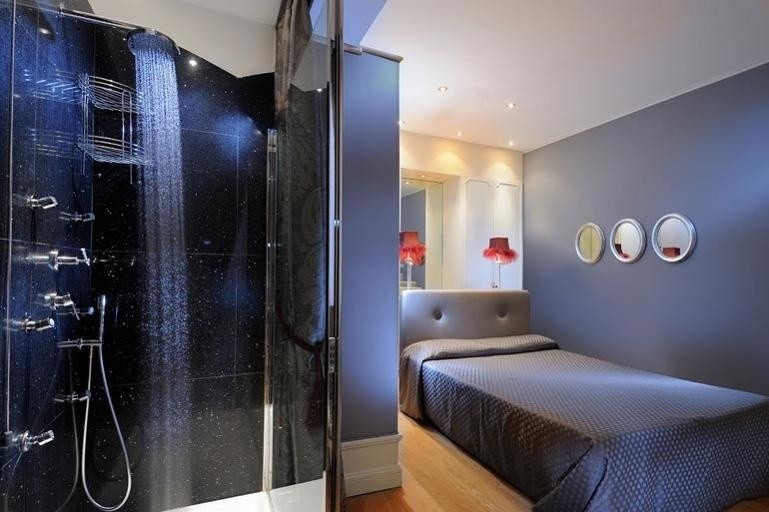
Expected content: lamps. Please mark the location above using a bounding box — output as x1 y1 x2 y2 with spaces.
399 229 432 289
484 233 519 288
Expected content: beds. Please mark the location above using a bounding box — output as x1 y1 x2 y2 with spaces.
398 288 769 512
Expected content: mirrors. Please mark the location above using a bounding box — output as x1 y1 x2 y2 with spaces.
609 217 647 264
401 180 441 288
651 212 699 264
574 222 605 266
